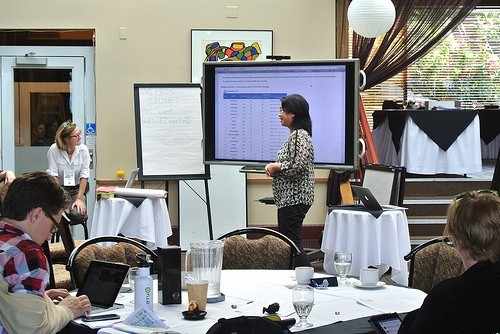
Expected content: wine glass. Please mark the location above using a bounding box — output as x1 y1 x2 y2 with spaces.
116 166 125 187
292 287 314 327
472 100 478 110
403 100 408 110
334 252 352 285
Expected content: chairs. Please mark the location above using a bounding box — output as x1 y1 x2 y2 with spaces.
66 236 159 289
405 238 466 294
326 169 355 206
46 212 88 289
213 225 301 270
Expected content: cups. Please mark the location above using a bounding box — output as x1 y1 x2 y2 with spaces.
185 279 208 311
128 267 138 286
181 271 188 290
360 268 379 286
412 104 418 109
295 267 315 285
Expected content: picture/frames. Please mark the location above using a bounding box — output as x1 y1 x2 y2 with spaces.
190 28 274 83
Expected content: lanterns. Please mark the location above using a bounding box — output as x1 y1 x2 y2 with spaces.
348 0 396 38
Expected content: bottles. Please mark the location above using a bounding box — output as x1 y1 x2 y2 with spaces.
425 101 428 110
134 255 153 313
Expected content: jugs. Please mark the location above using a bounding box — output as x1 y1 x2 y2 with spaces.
183 237 228 297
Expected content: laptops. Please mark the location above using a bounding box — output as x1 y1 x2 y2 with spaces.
77 259 131 319
351 185 409 210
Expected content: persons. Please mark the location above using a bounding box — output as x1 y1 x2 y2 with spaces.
206 316 296 334
48 119 90 223
31 119 59 146
265 94 315 270
0 170 91 334
397 190 500 334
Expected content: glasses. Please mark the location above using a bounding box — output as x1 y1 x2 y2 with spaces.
42 208 60 235
453 189 499 210
0 178 5 183
71 129 81 138
59 119 71 132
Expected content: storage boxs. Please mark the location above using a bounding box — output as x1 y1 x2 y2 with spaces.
157 246 181 305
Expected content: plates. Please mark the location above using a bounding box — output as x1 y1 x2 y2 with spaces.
352 280 386 288
182 309 207 319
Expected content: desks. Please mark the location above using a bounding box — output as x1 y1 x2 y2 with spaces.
90 197 174 249
437 108 500 159
55 269 429 334
372 110 482 178
321 206 411 287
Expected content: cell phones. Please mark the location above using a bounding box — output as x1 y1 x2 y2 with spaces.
82 314 119 322
367 311 403 334
